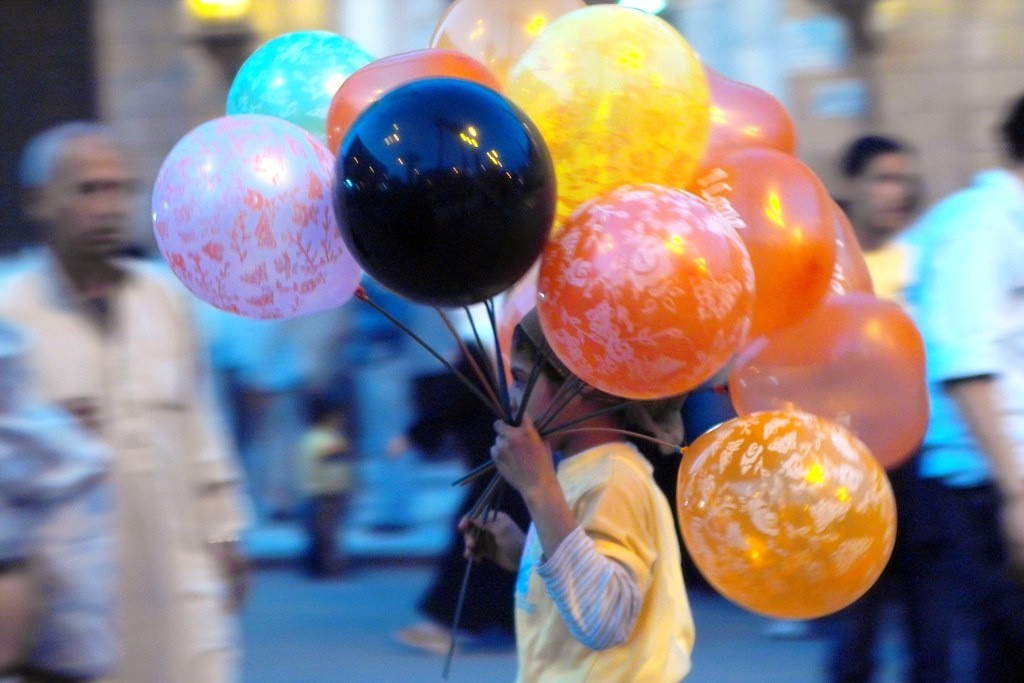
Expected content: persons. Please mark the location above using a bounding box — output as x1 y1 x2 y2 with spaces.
0 122 532 683
461 308 695 683
623 92 1023 683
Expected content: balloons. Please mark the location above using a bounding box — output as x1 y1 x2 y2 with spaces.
153 0 930 621
